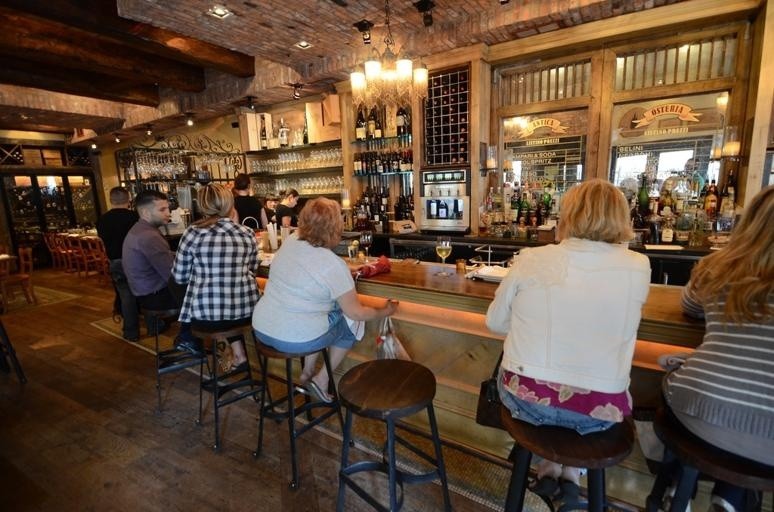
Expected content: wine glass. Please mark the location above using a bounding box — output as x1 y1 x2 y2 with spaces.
361 231 373 265
436 234 453 279
129 152 187 176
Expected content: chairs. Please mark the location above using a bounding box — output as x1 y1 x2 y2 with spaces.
0 220 123 393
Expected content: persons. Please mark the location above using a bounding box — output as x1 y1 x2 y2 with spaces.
484 179 653 509
261 193 280 229
276 187 300 233
505 172 515 184
170 183 262 374
652 184 774 512
96 186 163 342
121 190 206 356
619 158 705 199
250 196 399 405
232 173 263 229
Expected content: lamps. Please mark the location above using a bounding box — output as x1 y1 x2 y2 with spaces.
343 1 431 117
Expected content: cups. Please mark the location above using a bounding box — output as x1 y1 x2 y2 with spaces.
430 187 457 197
266 224 276 250
348 246 360 263
247 147 340 196
455 259 466 275
278 225 289 245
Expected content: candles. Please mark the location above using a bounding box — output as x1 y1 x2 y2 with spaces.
712 146 722 159
725 139 741 157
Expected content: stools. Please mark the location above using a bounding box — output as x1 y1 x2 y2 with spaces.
185 314 286 457
644 398 773 511
332 354 452 511
492 402 639 512
138 300 217 417
246 336 359 497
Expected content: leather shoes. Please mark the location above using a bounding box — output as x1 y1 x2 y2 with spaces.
173 335 207 357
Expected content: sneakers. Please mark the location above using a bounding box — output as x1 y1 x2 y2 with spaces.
661 477 693 512
710 483 746 512
147 324 168 336
122 333 140 342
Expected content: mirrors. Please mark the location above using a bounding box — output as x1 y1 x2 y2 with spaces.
499 91 594 197
603 76 734 209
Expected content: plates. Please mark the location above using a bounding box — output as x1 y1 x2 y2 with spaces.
473 266 509 283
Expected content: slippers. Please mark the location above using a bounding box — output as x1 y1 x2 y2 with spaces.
308 380 335 404
294 383 314 397
526 472 559 498
557 476 581 505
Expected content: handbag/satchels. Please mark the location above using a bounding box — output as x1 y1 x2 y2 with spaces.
473 348 514 432
374 299 412 363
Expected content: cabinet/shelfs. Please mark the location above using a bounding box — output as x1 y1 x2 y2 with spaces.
112 126 249 210
504 134 584 192
415 57 470 166
244 136 343 204
350 133 413 179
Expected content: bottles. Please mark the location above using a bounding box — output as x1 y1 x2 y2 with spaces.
261 118 266 149
352 184 388 234
302 112 308 144
352 148 412 175
429 199 463 220
354 104 411 141
278 118 289 147
479 181 559 242
394 183 414 222
625 170 735 248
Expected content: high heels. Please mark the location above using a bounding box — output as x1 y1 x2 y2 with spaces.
223 360 249 377
